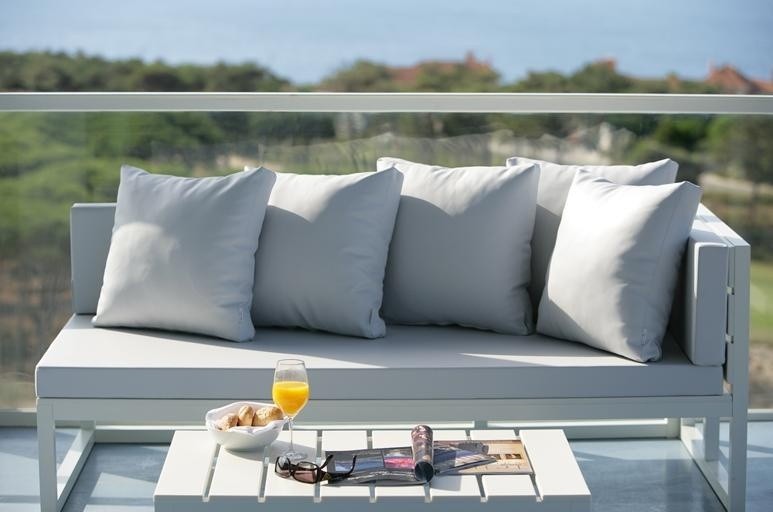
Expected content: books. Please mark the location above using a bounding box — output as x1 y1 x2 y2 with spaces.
433 441 532 475
432 442 496 475
325 426 433 486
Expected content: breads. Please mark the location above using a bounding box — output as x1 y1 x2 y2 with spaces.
219 405 283 431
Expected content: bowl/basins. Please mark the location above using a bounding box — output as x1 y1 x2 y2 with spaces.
206 399 285 453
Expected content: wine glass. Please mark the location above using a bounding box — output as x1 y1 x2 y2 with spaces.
271 358 309 460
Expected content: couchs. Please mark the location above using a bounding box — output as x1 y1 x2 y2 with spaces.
35 198 753 510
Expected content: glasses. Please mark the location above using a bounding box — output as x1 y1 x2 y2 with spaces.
275 455 357 484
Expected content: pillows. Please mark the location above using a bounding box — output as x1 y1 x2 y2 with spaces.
374 155 542 338
90 162 278 343
504 155 681 335
533 167 704 363
240 164 405 340
